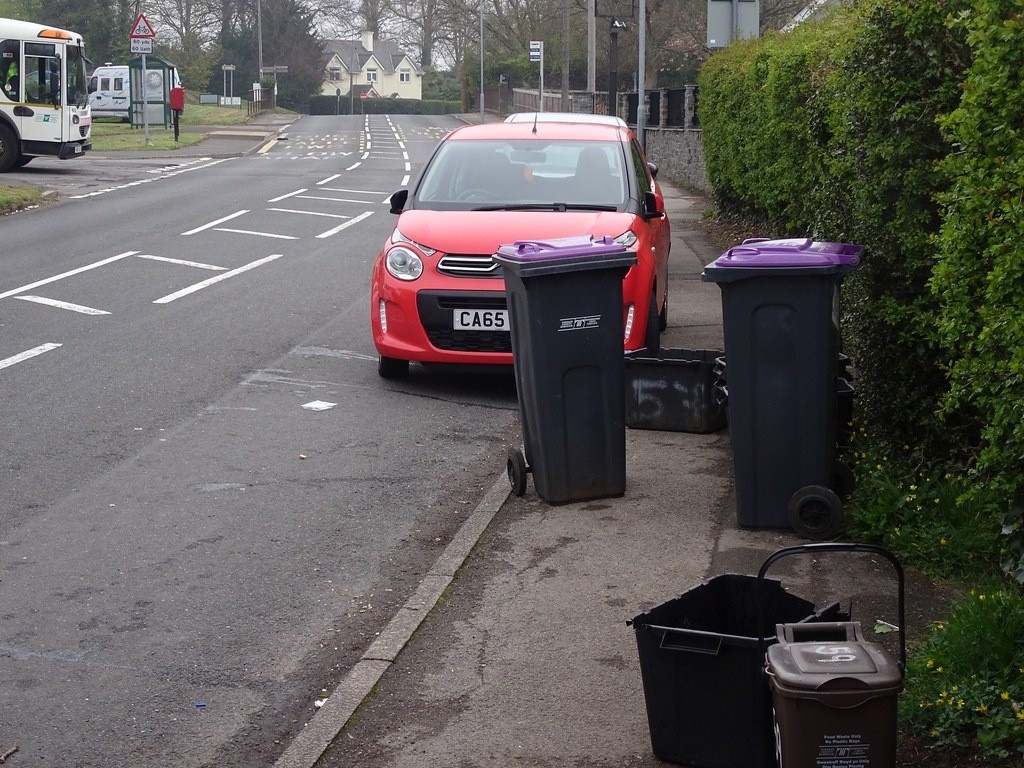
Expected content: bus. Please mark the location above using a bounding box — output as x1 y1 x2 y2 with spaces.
0 17 95 172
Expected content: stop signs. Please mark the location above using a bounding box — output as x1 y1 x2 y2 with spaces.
360 93 366 100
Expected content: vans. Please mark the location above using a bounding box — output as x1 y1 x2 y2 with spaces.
87 61 182 121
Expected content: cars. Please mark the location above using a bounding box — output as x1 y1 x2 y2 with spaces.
507 113 657 188
372 122 668 379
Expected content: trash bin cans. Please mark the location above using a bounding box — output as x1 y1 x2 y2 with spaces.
492 233 640 505
763 616 904 768
699 237 869 541
